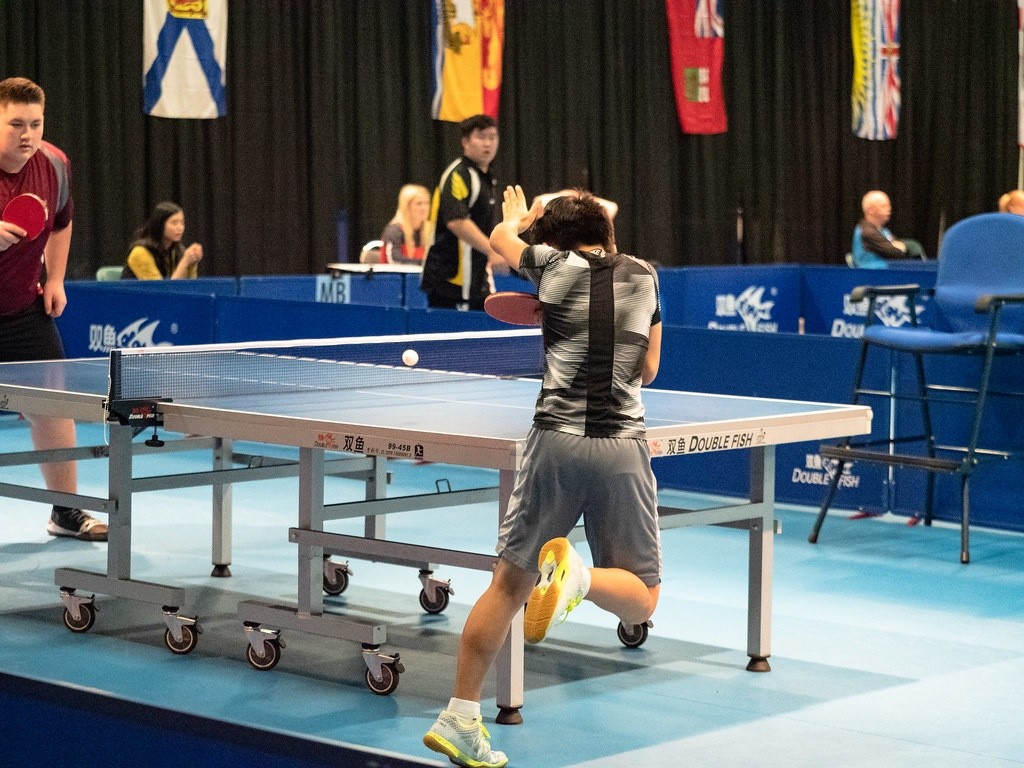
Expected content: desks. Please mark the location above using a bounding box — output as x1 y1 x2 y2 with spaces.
327 261 424 283
0 346 872 729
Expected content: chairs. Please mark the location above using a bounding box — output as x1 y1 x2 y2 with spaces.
359 240 386 263
809 211 1023 564
95 265 125 281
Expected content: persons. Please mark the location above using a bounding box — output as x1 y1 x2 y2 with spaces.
419 115 504 314
423 184 662 768
999 190 1024 215
851 190 907 269
381 184 431 265
120 201 203 281
0 75 110 541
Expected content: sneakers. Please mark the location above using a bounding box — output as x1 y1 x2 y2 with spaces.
523 538 585 645
423 710 509 768
47 506 109 541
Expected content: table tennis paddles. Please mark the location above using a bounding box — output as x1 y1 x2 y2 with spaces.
484 291 542 327
2 193 48 243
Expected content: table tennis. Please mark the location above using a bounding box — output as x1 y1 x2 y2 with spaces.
402 349 419 366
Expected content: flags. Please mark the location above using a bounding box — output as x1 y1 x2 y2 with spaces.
144 0 228 118
665 0 728 134
429 0 505 124
850 0 902 139
